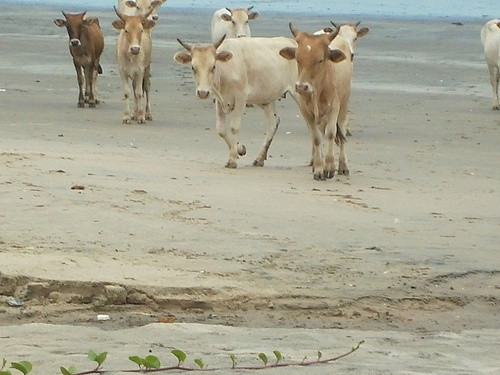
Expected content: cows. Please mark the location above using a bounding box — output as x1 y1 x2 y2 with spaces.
279 22 354 181
112 5 153 125
210 5 259 44
117 0 167 22
480 18 500 111
54 8 104 109
173 33 300 169
312 20 369 137
